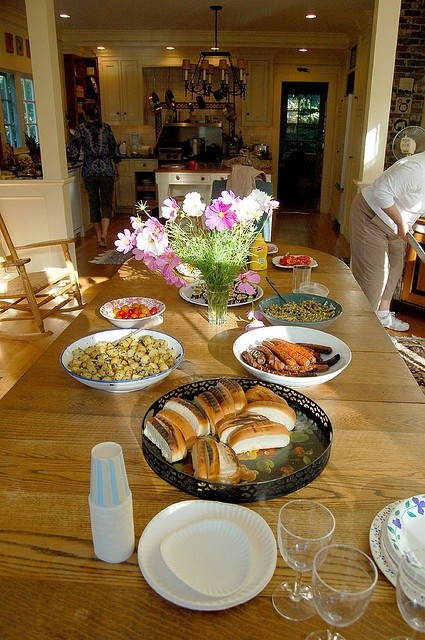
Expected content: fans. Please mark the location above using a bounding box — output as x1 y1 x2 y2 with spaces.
392 126 425 160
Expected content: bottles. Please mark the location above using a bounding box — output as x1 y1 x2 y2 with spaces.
249 232 268 271
215 118 219 123
213 118 216 123
172 109 178 123
205 115 210 124
178 109 185 123
205 161 209 168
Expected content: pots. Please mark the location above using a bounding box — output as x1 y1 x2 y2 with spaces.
222 93 238 122
252 143 270 160
148 76 163 114
184 136 209 158
165 76 176 112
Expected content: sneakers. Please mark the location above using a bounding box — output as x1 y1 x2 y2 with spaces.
377 312 410 332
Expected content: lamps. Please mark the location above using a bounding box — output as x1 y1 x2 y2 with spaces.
181 6 249 102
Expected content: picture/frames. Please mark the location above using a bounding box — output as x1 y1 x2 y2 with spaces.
26 39 31 58
5 32 14 53
16 35 24 55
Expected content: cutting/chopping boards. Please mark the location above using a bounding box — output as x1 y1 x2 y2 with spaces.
153 166 179 172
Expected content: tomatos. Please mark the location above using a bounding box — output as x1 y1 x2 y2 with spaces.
113 302 156 319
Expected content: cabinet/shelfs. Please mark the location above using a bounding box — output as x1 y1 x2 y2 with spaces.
241 54 275 127
116 160 157 207
99 57 143 127
157 173 271 242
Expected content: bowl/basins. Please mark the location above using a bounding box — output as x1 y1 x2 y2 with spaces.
259 293 343 330
233 326 353 388
59 328 184 393
299 281 330 299
99 296 167 329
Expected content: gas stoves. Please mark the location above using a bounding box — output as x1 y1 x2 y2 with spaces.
159 148 183 160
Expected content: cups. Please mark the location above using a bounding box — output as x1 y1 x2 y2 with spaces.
190 115 196 123
292 266 311 294
188 160 196 171
88 441 135 565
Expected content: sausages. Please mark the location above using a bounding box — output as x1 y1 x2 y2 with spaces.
242 338 340 376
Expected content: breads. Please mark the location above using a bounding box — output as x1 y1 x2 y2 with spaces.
224 421 291 453
218 413 267 441
217 442 243 484
210 386 236 417
163 397 210 437
216 378 247 414
156 408 196 448
194 391 227 432
190 440 220 482
242 400 303 431
143 415 187 462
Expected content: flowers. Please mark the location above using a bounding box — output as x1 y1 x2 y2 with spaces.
114 189 280 319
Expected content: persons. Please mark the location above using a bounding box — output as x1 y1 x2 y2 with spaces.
67 99 121 246
350 151 425 332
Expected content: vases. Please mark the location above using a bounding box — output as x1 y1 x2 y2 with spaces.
207 290 229 326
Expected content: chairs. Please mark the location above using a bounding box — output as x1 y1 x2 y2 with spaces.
0 212 88 341
211 180 272 242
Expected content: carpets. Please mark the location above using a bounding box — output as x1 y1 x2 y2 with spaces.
391 335 425 393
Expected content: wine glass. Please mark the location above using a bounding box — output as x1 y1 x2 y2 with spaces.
389 548 424 640
304 544 379 640
271 498 336 620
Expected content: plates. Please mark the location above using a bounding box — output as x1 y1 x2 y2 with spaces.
137 499 278 612
178 279 264 308
272 255 319 269
382 527 400 574
176 263 204 277
369 497 408 589
388 494 425 577
266 242 279 255
143 196 155 200
406 232 425 264
160 518 252 598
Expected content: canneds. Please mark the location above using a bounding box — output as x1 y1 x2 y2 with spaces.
130 131 139 145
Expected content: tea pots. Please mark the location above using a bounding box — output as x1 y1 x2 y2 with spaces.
119 140 127 156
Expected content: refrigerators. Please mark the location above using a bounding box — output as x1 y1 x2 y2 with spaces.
332 93 353 225
